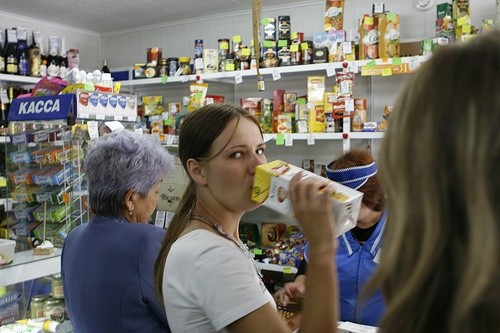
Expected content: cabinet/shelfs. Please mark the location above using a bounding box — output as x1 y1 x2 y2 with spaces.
0 65 429 332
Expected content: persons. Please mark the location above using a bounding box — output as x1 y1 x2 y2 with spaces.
273 149 386 327
60 130 172 333
358 27 500 333
152 102 339 333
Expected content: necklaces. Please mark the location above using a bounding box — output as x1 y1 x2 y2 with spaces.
192 214 263 277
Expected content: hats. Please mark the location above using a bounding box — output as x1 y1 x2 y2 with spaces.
325 158 377 190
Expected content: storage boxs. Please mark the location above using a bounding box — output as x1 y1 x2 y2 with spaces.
0 126 87 238
136 90 336 134
34 244 54 255
134 63 159 78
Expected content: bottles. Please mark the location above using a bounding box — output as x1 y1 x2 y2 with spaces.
0 26 69 81
100 59 110 73
